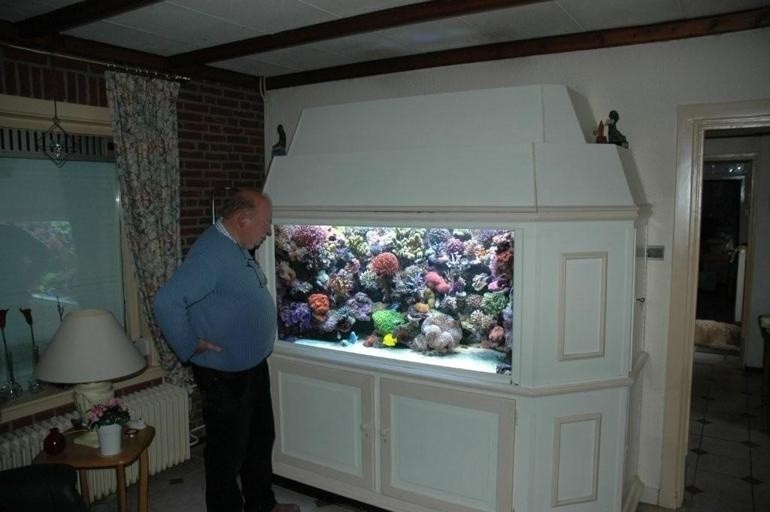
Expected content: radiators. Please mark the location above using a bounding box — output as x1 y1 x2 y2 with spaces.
0 383 191 504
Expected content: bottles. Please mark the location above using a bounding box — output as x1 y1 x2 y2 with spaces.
44 428 66 457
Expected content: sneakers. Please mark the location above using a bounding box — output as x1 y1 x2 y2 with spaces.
247 501 301 512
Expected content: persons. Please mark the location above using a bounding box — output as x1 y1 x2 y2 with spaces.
152 185 303 512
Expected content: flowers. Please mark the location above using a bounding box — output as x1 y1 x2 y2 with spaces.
0 296 73 400
84 397 132 432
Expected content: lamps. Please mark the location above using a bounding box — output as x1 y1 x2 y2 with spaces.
35 308 148 447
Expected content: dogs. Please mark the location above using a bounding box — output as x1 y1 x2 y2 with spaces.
695 319 742 352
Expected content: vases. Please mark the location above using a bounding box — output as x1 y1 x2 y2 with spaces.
94 423 123 456
